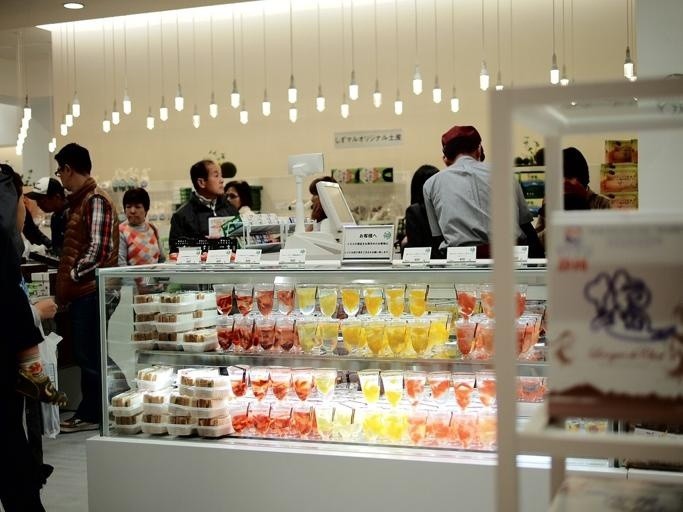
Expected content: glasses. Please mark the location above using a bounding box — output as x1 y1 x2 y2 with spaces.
225 193 238 200
55 167 63 177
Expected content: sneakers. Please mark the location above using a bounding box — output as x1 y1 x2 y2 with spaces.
18 374 67 404
60 415 100 433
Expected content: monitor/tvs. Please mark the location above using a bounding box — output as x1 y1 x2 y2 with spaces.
314 182 357 233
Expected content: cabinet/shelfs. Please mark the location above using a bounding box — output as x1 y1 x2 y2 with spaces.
487 74 682 511
84 263 616 511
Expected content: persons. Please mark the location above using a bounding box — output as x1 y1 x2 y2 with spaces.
537 147 613 251
309 175 341 221
0 143 165 512
224 181 258 220
404 163 440 257
423 124 543 258
168 159 244 254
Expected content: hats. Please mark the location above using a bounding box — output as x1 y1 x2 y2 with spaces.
442 126 485 167
24 176 63 200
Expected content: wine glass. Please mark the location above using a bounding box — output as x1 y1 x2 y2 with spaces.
212 283 543 359
227 364 497 449
